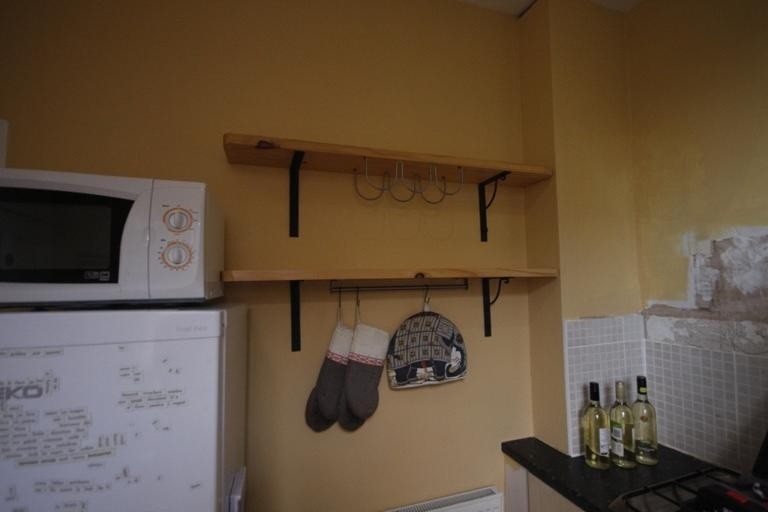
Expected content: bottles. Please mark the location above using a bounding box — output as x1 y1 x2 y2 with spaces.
581 375 658 470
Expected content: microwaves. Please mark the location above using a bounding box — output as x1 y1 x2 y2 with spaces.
0 167 225 309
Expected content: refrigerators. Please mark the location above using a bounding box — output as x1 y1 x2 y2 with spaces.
0 303 248 512
